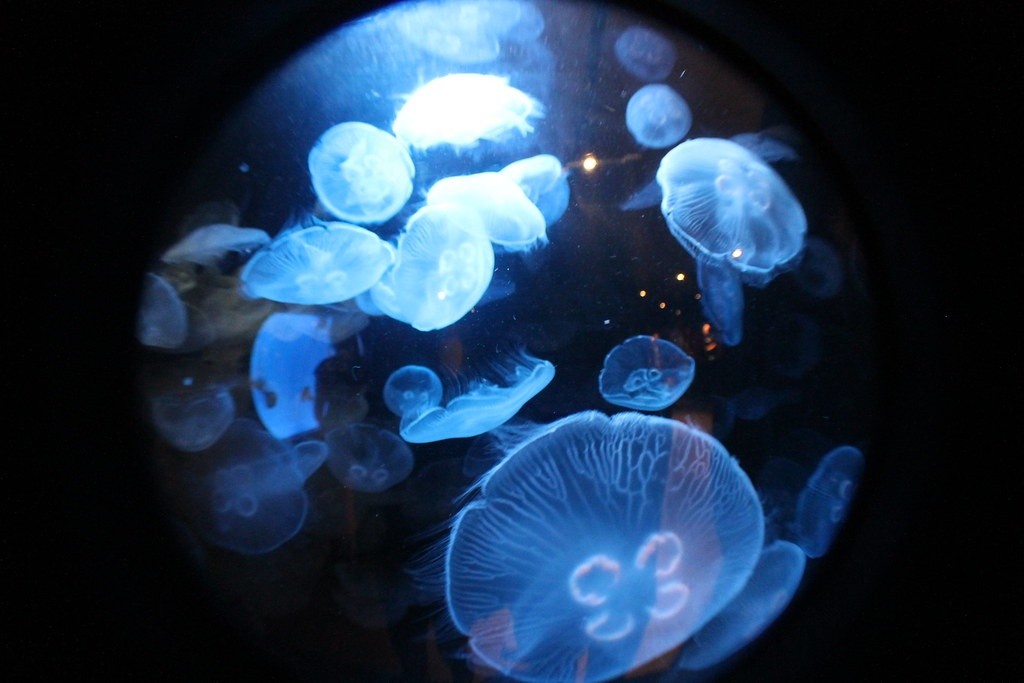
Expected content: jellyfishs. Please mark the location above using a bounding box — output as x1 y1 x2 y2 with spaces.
130 22 865 683
